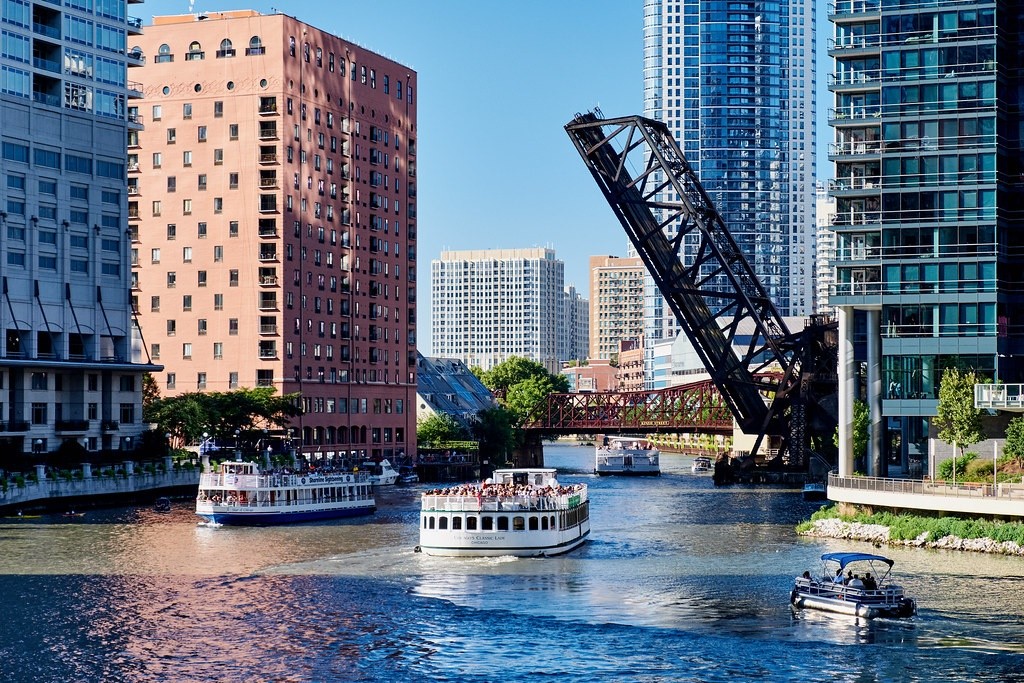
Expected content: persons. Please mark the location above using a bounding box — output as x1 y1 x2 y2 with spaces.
803 568 877 594
419 450 464 463
426 478 580 509
889 377 901 399
598 444 653 451
513 519 524 530
196 463 359 506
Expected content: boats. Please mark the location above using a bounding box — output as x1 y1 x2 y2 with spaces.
789 552 918 620
364 453 420 486
800 482 827 501
194 460 377 526
593 438 663 476
693 454 715 474
414 468 591 558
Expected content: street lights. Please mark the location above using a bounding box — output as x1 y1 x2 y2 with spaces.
235 429 240 453
37 439 42 453
202 432 208 455
125 436 131 450
263 429 269 449
83 437 89 450
